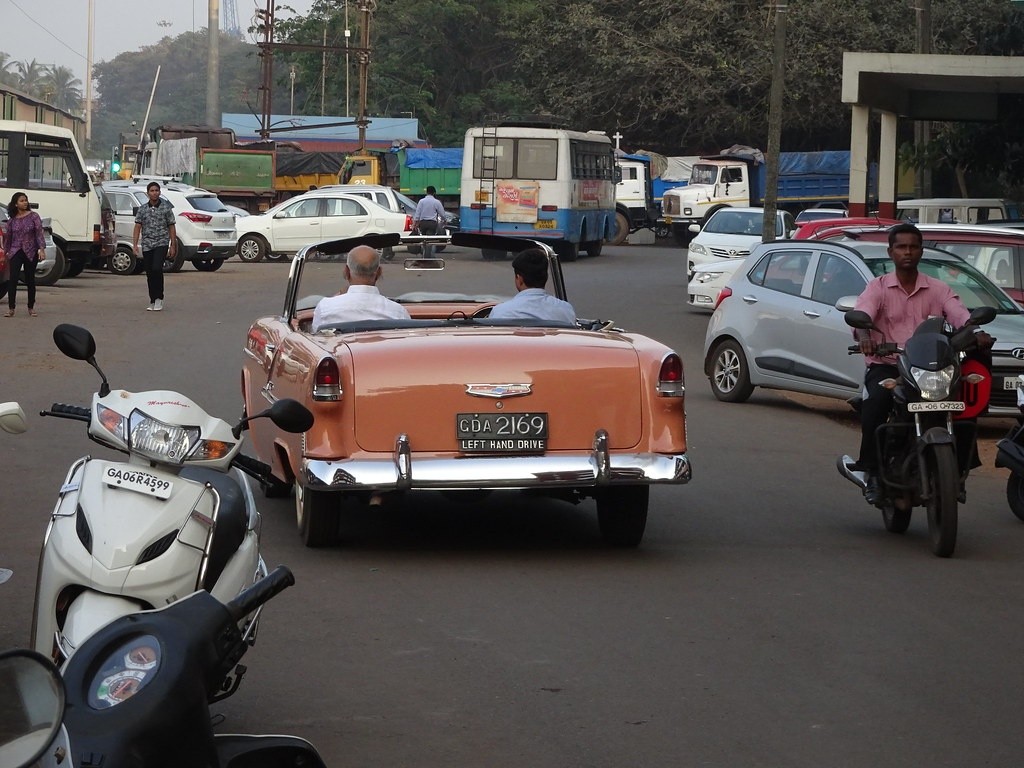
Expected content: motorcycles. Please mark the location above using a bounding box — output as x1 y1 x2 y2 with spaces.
995 376 1024 517
836 305 998 555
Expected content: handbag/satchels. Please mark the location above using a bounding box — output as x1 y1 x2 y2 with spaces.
407 228 422 255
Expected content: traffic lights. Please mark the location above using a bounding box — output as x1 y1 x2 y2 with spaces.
111 155 121 174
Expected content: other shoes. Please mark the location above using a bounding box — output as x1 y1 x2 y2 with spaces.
154 298 163 311
28 308 38 317
4 308 15 317
146 303 155 311
956 478 966 504
865 474 888 504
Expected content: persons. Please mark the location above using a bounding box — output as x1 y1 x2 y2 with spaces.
412 186 447 258
3 192 46 317
133 182 177 311
848 225 983 512
487 249 577 326
312 246 410 333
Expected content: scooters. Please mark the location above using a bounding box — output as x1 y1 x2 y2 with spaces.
0 324 326 767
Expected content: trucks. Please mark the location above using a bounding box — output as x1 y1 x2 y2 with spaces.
0 120 102 287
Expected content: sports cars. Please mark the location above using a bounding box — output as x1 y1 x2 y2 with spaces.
242 231 692 548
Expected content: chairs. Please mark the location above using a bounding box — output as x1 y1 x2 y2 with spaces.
314 317 576 334
996 259 1009 284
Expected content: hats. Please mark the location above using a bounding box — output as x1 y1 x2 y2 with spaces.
511 249 549 271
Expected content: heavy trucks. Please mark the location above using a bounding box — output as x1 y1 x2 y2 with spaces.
655 151 880 249
114 127 345 215
338 145 465 214
603 150 701 246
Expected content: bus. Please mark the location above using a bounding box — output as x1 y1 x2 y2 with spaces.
460 115 616 261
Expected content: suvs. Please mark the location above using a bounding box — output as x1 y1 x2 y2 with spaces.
132 174 239 272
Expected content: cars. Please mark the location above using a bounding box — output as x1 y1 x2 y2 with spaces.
93 180 174 276
704 241 1024 417
687 206 1024 311
235 184 460 263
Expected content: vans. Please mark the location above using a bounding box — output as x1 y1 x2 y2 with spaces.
896 198 1020 225
